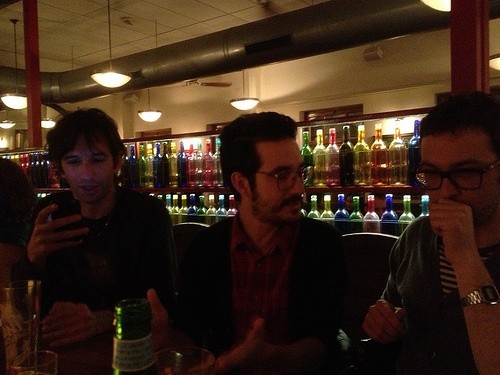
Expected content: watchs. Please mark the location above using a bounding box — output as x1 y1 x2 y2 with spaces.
461 285 500 304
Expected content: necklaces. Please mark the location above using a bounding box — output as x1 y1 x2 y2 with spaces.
96 205 112 237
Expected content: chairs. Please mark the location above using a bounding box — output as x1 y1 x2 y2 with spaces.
171 222 400 321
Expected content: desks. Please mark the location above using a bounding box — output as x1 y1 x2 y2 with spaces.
0 264 206 375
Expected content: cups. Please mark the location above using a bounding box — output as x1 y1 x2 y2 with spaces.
154 345 216 375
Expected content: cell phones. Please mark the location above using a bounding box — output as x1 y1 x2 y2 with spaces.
51 199 81 240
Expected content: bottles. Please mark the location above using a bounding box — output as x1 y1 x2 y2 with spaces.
1 120 430 237
111 297 155 375
0 279 57 375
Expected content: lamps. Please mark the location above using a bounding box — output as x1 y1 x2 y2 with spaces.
0 0 262 130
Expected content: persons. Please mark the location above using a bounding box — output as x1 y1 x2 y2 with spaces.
0 157 34 305
173 111 349 375
361 91 500 375
10 108 178 348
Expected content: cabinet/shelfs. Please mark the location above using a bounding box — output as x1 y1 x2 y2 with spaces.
0 106 437 194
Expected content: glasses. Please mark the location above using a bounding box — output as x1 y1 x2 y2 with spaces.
413 158 500 191
241 167 312 190
